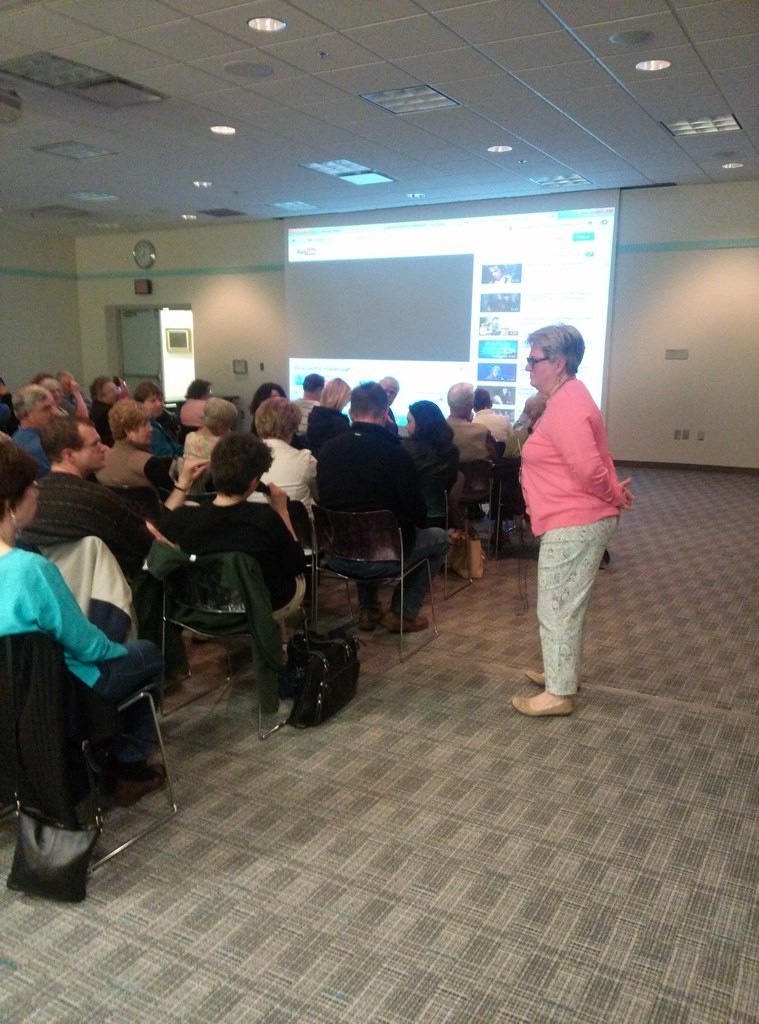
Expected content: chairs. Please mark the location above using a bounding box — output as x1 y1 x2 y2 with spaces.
0 439 523 878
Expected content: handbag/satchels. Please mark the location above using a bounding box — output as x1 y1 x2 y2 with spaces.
7 806 101 903
287 632 359 727
446 528 484 578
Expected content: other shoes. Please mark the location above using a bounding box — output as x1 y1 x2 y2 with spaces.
468 506 486 519
503 521 517 532
380 612 429 631
512 692 573 716
358 603 388 630
525 671 581 691
598 550 610 568
163 655 191 681
117 763 166 807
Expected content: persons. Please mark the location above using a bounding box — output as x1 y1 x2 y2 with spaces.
14 413 153 574
154 430 306 665
0 369 549 487
315 381 451 633
0 440 168 808
246 396 320 548
512 326 635 716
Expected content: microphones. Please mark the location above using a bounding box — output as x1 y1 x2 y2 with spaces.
254 481 292 505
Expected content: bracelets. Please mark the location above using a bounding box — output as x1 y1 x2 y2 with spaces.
174 485 185 492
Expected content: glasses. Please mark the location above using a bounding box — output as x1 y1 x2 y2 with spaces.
527 356 548 367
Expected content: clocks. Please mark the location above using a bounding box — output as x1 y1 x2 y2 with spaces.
132 239 156 268
